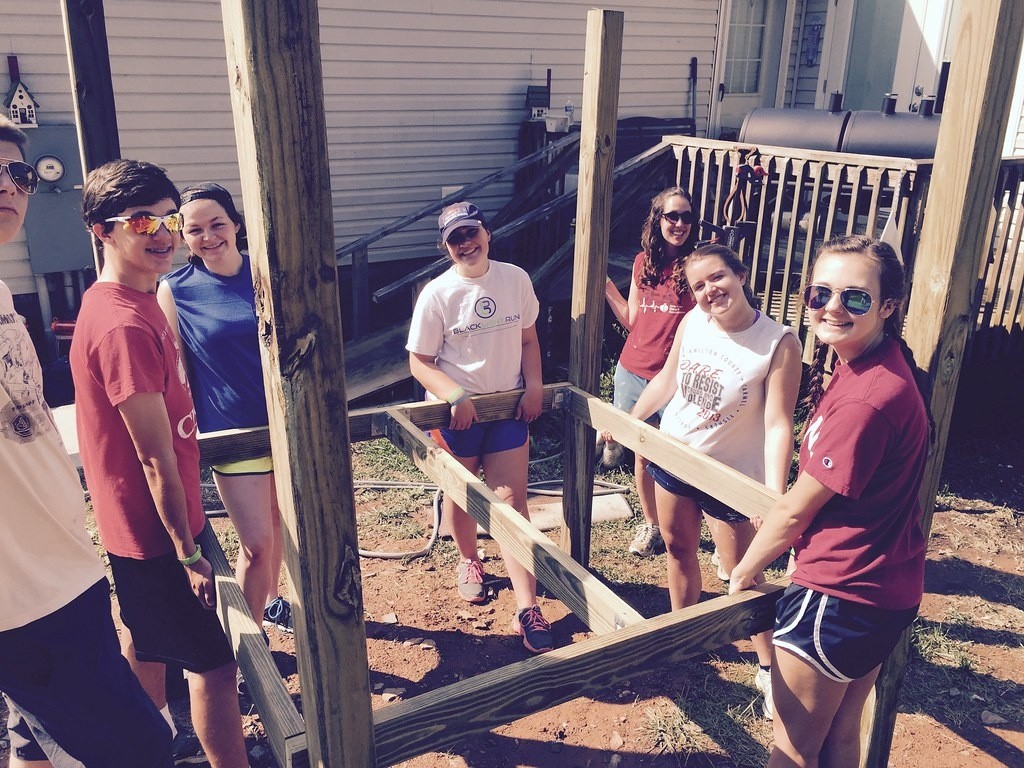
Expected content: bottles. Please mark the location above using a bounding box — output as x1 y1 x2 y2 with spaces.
565 99 574 125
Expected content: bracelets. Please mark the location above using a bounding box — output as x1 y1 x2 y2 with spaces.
447 387 464 403
452 392 467 406
179 545 202 565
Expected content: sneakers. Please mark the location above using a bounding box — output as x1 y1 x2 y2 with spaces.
238 633 269 694
457 557 485 601
168 729 199 765
261 594 295 632
755 666 778 722
627 520 662 558
712 549 730 583
511 606 554 653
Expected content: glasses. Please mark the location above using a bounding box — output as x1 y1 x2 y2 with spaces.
802 285 893 317
92 213 185 237
660 211 693 225
0 156 41 195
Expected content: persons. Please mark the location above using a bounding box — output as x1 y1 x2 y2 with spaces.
405 201 552 652
600 244 805 720
0 109 176 768
728 236 936 768
606 187 695 559
68 158 250 768
154 184 291 710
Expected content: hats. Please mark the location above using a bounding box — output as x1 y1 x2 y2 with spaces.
438 202 485 243
179 182 247 238
